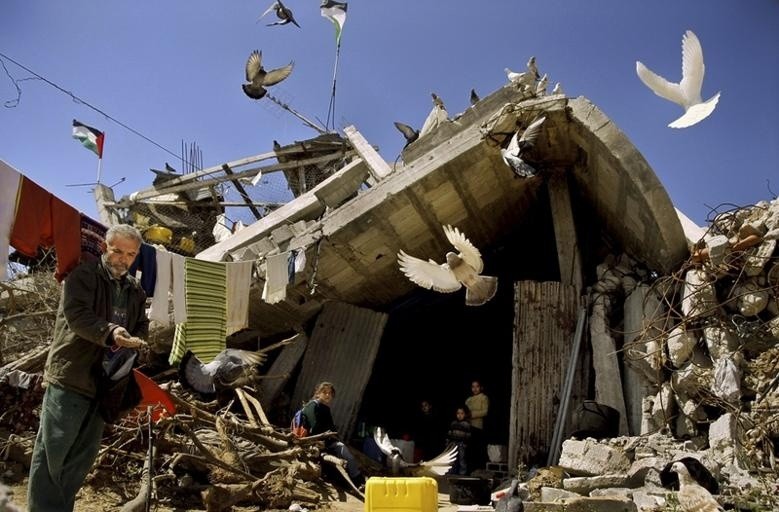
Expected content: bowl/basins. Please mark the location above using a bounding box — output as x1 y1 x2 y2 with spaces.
447 476 492 506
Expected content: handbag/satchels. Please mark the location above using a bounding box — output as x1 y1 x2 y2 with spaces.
290 399 320 437
89 363 143 425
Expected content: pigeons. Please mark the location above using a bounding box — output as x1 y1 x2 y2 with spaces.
494 479 526 512
469 87 480 103
393 120 421 149
633 28 724 133
240 48 297 102
395 222 500 308
176 346 269 404
503 55 562 97
258 0 301 31
370 423 461 478
430 91 447 112
498 115 548 182
669 461 727 512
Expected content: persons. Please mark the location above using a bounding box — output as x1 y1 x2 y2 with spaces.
292 381 494 494
27 223 148 512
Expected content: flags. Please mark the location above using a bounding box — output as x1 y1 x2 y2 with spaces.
72 121 105 161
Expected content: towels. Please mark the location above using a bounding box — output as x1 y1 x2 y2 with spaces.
261 253 289 305
225 260 250 336
